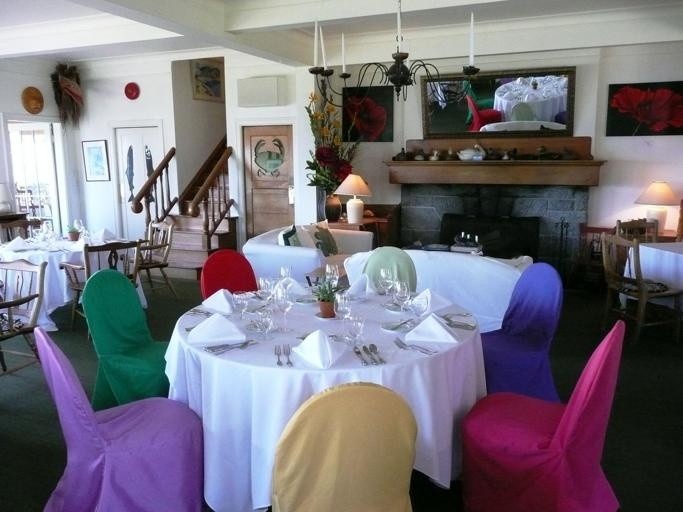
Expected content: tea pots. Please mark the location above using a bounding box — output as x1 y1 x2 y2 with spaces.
458 144 485 161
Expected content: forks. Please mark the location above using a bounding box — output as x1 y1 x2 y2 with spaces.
283 343 294 368
273 343 283 367
390 336 438 356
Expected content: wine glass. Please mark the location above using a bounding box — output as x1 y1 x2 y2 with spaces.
232 263 428 351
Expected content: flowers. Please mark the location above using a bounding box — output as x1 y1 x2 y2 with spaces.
303 85 359 197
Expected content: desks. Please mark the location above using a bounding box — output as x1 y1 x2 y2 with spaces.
337 218 389 252
176 280 486 502
346 248 521 334
620 241 682 308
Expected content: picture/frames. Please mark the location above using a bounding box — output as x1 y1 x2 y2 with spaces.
82 139 112 184
188 57 224 103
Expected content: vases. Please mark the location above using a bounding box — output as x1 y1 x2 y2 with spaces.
324 193 341 223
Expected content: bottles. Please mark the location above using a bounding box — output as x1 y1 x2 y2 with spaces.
459 231 481 247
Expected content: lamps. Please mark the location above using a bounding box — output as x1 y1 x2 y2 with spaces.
632 179 679 233
332 171 371 226
298 0 480 119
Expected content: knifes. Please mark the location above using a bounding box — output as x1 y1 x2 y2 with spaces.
351 344 367 367
362 345 378 364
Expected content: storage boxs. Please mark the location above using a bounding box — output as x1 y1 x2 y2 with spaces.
235 72 297 109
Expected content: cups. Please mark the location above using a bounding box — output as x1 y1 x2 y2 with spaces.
29 218 92 250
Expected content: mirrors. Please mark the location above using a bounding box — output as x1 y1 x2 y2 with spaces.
416 62 578 140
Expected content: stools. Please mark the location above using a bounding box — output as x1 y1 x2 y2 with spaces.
0 185 174 378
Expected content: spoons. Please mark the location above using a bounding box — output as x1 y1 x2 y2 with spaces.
368 343 387 363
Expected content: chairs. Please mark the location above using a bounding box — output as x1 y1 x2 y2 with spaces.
267 380 418 512
615 221 658 266
595 232 679 347
462 320 630 510
238 224 321 284
424 76 568 131
77 268 171 403
200 249 259 300
480 263 565 402
361 245 419 294
27 323 203 511
